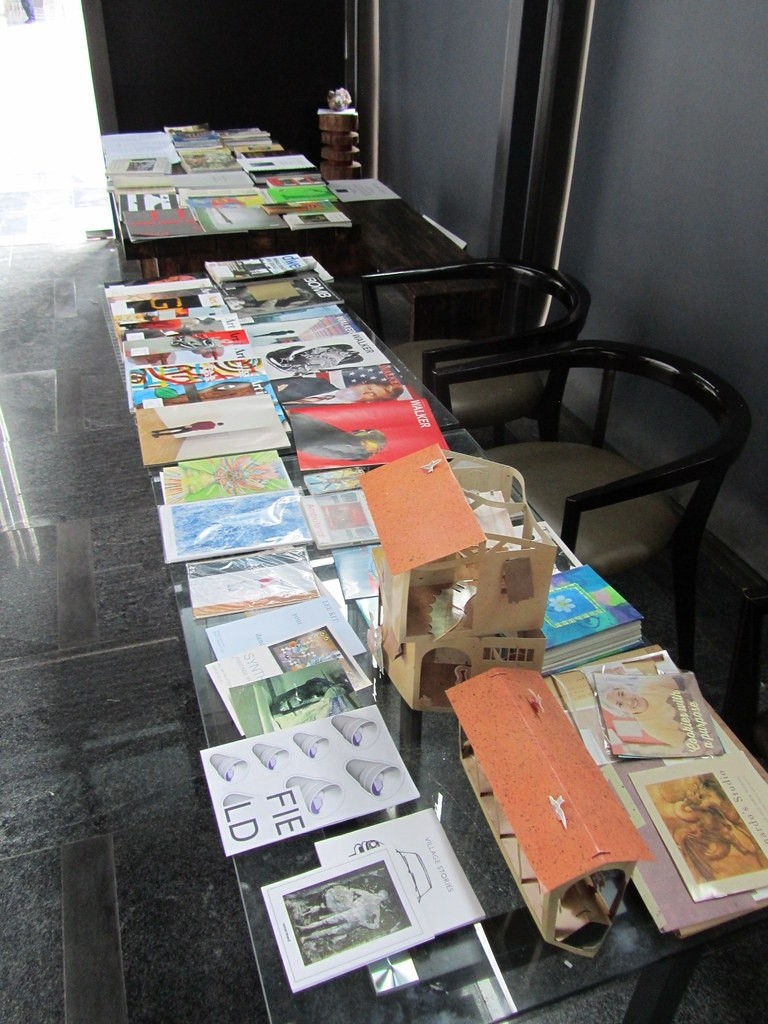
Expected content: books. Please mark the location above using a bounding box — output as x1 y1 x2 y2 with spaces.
104 253 768 995
101 124 352 244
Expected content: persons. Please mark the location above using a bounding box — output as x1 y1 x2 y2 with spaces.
270 376 402 404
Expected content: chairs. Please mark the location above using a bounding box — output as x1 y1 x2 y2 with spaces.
431 341 753 673
359 258 590 440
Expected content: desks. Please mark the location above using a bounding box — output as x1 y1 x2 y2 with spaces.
101 254 768 1024
99 132 363 281
